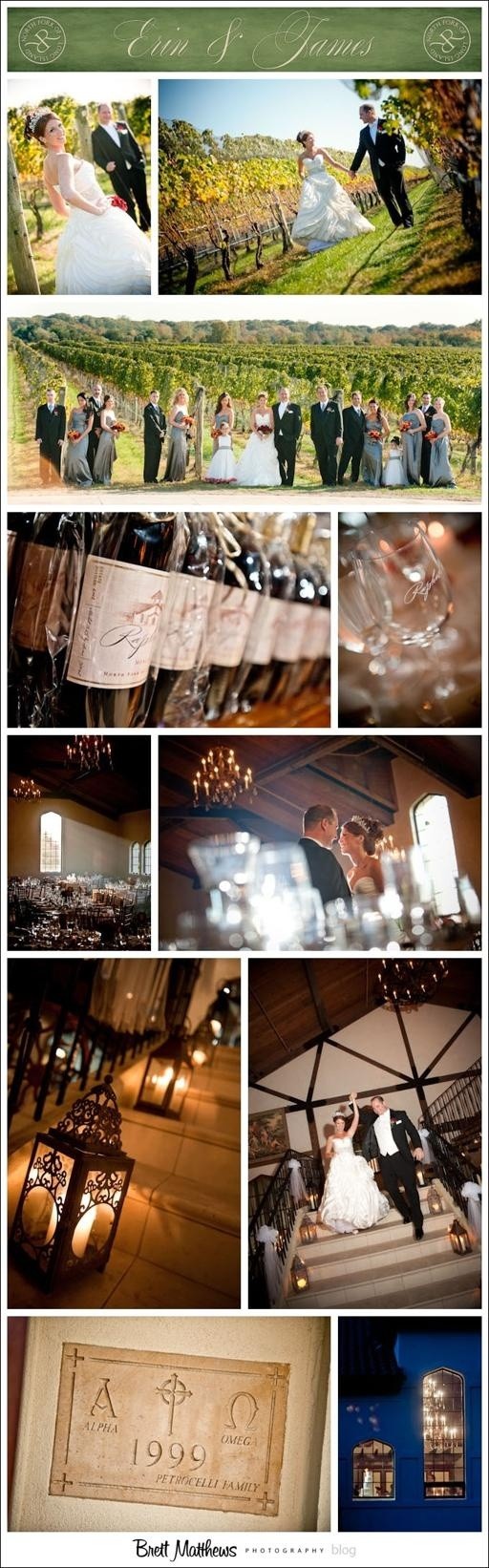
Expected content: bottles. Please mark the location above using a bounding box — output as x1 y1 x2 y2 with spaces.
18 515 330 726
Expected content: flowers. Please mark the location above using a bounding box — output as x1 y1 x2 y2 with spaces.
108 195 129 212
210 429 220 438
54 407 58 416
257 424 273 437
66 430 81 441
110 422 126 432
180 415 194 425
376 126 383 135
423 430 438 440
396 420 415 437
366 429 382 441
390 1116 397 1130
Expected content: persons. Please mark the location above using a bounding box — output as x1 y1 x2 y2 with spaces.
337 814 385 913
35 383 456 487
91 103 151 232
290 129 376 256
348 103 415 229
317 1093 390 1236
349 1092 425 1241
288 803 354 943
22 106 150 295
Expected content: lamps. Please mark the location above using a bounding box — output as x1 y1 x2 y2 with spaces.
133 1023 195 1120
62 735 115 783
375 958 450 1005
413 1162 473 1256
290 1181 320 1294
7 1075 134 1296
12 777 41 804
192 1019 218 1069
192 742 259 813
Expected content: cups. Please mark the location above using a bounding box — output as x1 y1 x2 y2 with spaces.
191 831 482 955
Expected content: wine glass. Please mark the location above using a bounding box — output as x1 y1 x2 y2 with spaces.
337 519 473 719
22 868 150 947
